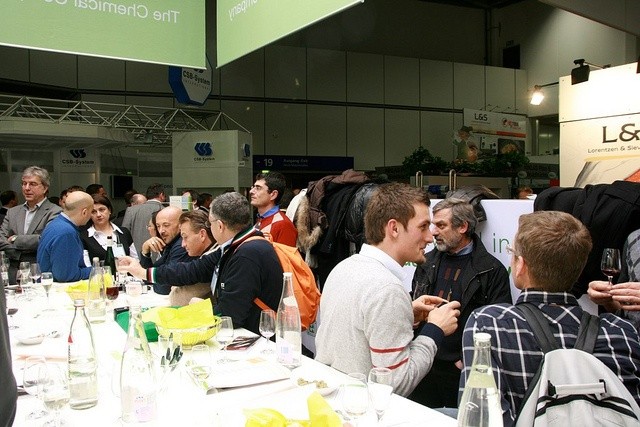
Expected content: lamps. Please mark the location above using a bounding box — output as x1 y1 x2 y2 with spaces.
571 58 589 87
531 85 545 106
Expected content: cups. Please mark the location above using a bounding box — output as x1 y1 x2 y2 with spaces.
158 331 183 359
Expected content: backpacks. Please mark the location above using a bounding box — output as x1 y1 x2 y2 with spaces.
511 303 637 427
235 236 320 332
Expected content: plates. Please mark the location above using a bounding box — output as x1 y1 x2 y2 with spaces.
291 366 342 397
14 324 65 346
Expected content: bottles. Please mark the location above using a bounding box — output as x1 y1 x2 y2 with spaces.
67 298 99 411
275 272 302 370
87 257 107 325
119 305 157 425
0 251 9 288
455 333 504 427
103 235 117 287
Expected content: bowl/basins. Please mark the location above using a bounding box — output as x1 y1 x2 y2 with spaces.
67 289 109 307
155 315 222 351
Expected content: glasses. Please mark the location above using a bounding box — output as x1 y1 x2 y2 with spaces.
250 185 262 191
506 244 517 256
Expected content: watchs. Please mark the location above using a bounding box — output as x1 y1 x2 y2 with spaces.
12 234 18 242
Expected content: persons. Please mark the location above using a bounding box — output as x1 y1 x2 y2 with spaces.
179 208 218 257
1 190 19 225
139 205 198 269
181 190 203 209
147 208 162 238
116 192 284 339
0 165 64 283
199 193 213 208
587 228 639 335
122 183 168 256
117 188 139 216
311 181 462 396
457 210 639 427
86 184 108 198
66 185 84 197
113 193 148 227
250 170 298 247
79 193 140 268
407 198 513 408
59 189 67 208
512 184 533 199
36 190 100 282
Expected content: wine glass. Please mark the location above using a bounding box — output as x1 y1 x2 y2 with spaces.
16 270 29 300
20 272 33 303
215 316 234 361
36 363 71 427
30 264 42 290
106 285 120 313
41 272 54 303
115 255 133 281
259 310 276 354
20 262 31 285
343 373 371 427
187 345 213 403
368 368 394 427
22 356 51 420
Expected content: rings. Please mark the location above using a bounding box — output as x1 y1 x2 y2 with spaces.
152 241 155 245
155 240 158 243
